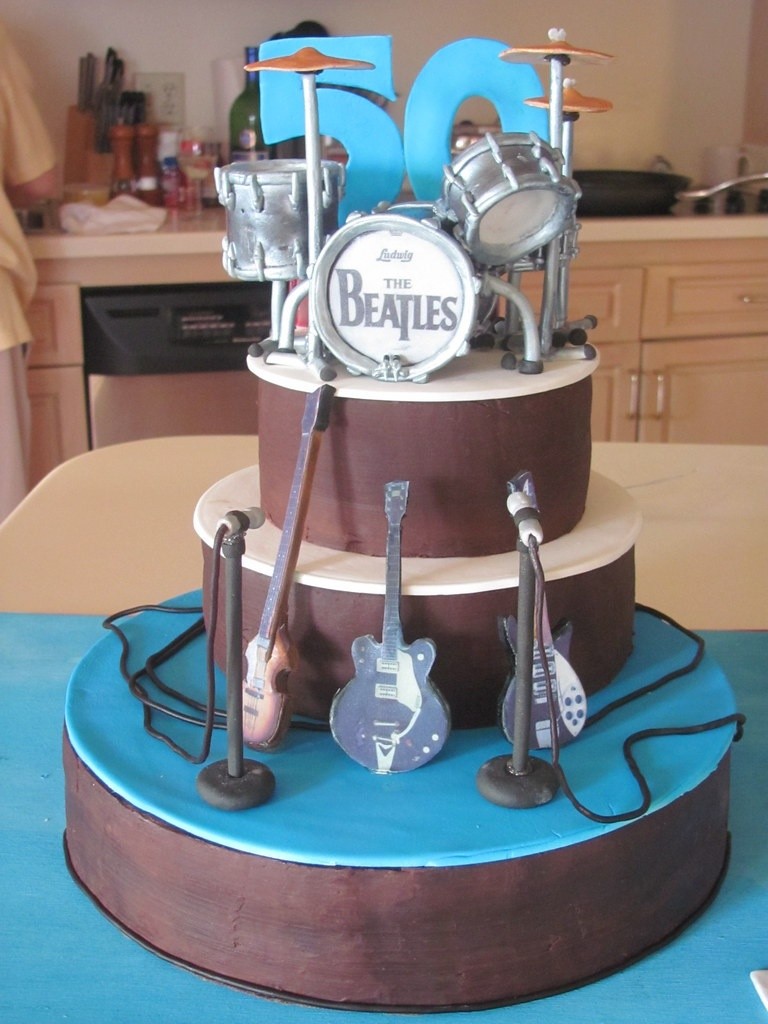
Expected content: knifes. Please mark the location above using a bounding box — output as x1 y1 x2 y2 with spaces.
79 47 146 155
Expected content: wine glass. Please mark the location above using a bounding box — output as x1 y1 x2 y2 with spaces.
176 126 218 218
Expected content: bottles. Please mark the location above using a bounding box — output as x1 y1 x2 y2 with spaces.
228 45 277 163
161 156 200 209
108 126 136 200
136 124 161 209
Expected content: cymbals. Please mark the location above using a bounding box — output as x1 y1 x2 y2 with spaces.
521 85 614 114
241 47 378 74
498 39 613 67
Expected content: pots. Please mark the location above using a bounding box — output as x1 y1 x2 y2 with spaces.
573 170 768 217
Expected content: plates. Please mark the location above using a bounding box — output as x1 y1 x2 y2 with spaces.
58 205 167 235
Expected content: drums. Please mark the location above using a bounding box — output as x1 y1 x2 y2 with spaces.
434 131 584 266
215 156 347 282
310 213 481 385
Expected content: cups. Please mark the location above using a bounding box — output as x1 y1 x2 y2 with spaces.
64 185 108 208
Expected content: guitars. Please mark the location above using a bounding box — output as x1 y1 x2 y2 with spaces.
498 469 590 753
329 478 453 775
241 385 339 750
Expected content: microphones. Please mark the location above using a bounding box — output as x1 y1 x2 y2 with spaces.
216 506 265 539
507 492 543 547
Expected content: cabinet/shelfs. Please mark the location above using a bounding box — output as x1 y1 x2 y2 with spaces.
495 236 768 446
20 282 91 495
